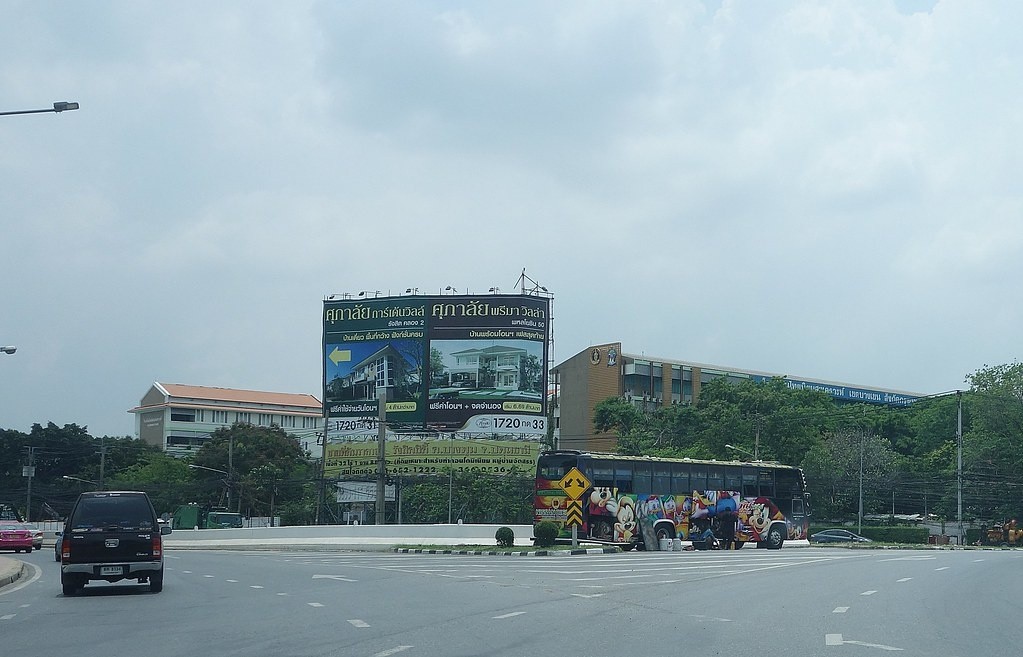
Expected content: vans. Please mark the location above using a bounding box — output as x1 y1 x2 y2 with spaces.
54 491 172 594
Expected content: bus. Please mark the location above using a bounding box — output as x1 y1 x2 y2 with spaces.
532 449 814 550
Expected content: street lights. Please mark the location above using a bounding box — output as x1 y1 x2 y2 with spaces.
845 416 863 533
724 445 759 460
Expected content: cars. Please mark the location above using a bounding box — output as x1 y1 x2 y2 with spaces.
810 530 873 544
0 520 43 553
452 382 465 387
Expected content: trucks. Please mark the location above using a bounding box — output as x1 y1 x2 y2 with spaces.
171 504 243 530
